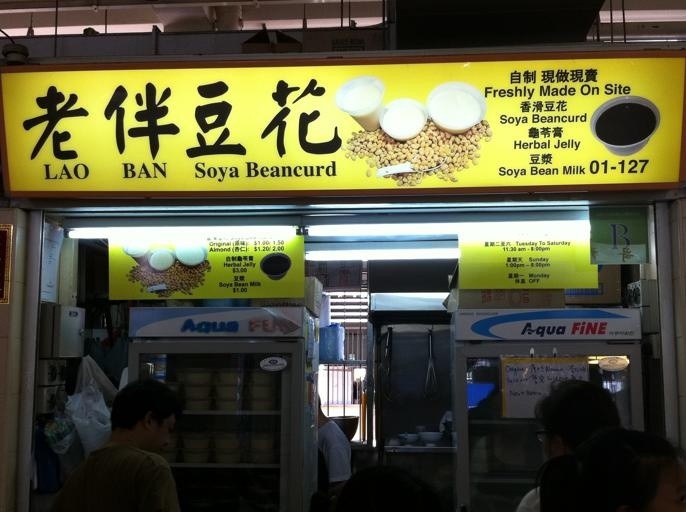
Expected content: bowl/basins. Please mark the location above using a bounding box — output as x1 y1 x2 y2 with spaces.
588 94 661 155
399 431 443 446
260 252 292 280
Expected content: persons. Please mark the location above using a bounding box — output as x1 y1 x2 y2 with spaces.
334 464 455 512
46 379 184 512
318 396 352 512
516 380 622 512
535 426 686 512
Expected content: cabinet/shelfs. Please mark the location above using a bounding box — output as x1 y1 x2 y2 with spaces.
117 307 318 509
448 309 646 512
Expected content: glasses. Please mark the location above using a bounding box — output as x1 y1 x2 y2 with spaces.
534 427 550 444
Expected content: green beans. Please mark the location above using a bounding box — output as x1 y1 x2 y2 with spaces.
125 259 211 297
341 121 494 188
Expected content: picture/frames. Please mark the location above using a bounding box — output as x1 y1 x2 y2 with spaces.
0 223 13 304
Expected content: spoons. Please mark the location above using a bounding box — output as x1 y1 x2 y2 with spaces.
376 149 447 180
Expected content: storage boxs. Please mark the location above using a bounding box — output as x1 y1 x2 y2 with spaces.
315 294 331 327
320 326 345 361
446 263 566 310
248 276 324 318
564 263 622 304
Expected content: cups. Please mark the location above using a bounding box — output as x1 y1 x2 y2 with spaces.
334 75 488 141
124 243 207 270
